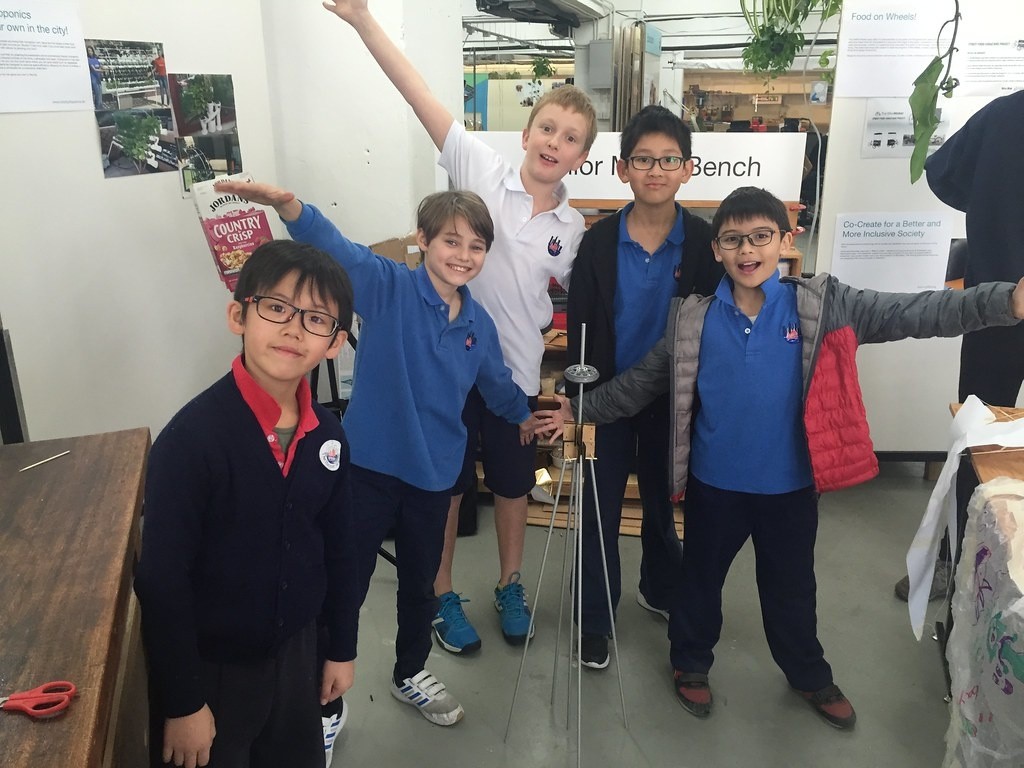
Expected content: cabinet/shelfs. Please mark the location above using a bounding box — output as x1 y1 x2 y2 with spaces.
108 135 178 173
96 48 160 110
475 248 802 498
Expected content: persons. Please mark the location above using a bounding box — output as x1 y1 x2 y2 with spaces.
323 0 597 655
151 50 169 107
534 185 1024 732
133 239 362 768
215 180 551 767
894 88 1024 602
566 105 725 670
800 120 810 131
87 46 103 110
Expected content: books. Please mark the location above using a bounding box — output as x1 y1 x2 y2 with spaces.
189 171 273 293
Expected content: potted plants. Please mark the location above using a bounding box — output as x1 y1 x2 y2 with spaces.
107 77 160 168
181 77 228 134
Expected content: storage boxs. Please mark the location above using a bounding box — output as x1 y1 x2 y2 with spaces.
370 235 421 270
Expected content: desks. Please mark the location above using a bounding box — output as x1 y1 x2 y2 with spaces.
0 427 153 768
936 403 1024 699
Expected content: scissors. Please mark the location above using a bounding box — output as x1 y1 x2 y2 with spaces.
0 681 75 717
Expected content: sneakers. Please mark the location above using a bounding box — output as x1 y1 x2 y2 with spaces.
390 660 464 725
577 631 612 668
673 666 713 717
432 591 481 654
894 559 957 603
494 571 535 647
637 589 670 620
321 698 349 768
796 680 856 729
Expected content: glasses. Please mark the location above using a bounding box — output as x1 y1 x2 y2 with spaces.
245 295 345 337
714 229 787 250
626 156 687 171
